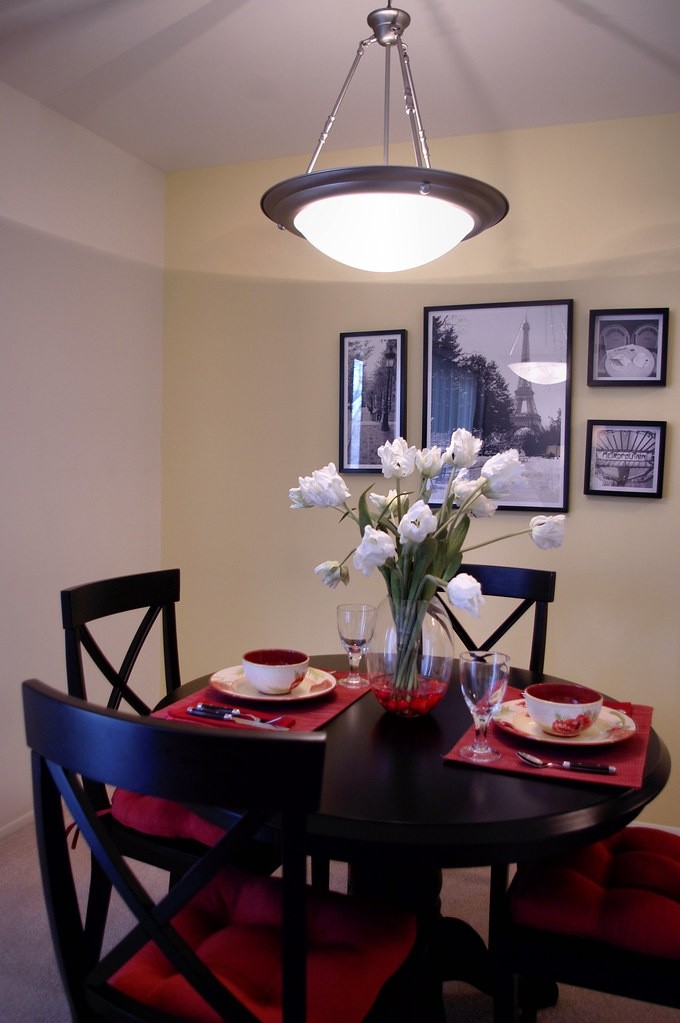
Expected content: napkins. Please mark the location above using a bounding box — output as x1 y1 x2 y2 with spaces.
444 685 654 791
149 671 378 734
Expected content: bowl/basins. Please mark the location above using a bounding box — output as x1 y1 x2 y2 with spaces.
525 683 603 737
242 649 310 696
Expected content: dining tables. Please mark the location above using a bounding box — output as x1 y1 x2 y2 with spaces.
92 656 674 992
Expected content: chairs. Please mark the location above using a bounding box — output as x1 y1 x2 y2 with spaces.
492 826 680 1023
20 679 443 1023
311 564 555 891
61 568 328 962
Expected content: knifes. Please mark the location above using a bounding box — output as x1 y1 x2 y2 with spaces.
186 707 290 731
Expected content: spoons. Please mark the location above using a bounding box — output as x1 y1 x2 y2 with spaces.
516 751 616 774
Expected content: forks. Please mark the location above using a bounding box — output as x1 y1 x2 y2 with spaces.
198 703 283 724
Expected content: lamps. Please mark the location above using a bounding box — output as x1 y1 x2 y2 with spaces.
506 306 566 385
261 0 508 273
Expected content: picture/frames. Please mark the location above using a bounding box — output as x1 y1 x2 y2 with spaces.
339 329 408 473
421 299 575 513
584 419 667 499
586 307 670 386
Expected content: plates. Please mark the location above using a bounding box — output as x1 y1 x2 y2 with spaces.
605 345 655 377
492 699 637 745
210 665 336 701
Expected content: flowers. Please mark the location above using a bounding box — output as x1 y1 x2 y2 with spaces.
289 429 566 698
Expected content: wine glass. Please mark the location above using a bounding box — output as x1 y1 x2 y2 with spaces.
459 652 510 762
336 603 375 689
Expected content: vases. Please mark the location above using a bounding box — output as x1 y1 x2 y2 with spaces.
358 597 455 715
